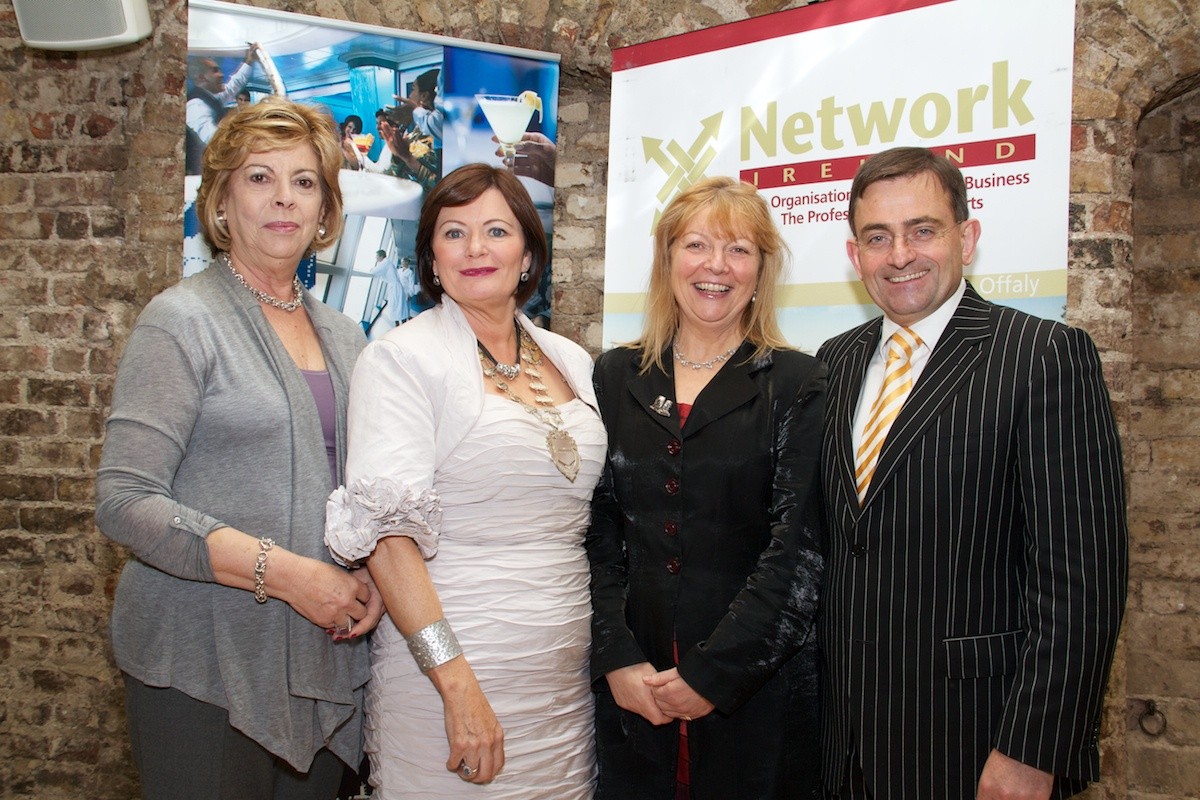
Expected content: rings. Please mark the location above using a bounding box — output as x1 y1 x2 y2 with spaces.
461 763 478 776
686 717 691 720
336 618 354 636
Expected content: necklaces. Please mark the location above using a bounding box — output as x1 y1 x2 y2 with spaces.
672 341 735 368
223 251 304 312
476 322 582 483
477 318 521 380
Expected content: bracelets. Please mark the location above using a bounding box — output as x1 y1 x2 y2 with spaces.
407 618 465 673
255 536 274 603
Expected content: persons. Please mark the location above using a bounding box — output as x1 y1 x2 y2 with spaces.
321 163 613 800
584 178 827 800
186 42 443 195
372 250 408 326
398 258 417 317
813 146 1131 800
96 93 389 800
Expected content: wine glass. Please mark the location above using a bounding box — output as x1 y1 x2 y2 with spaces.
351 134 375 173
474 94 534 174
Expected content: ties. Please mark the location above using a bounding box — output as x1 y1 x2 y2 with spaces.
854 326 925 507
